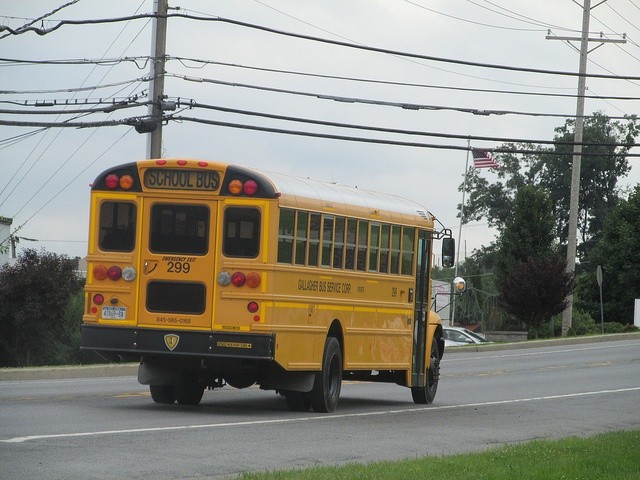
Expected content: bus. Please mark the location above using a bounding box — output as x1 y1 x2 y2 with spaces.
80 157 468 411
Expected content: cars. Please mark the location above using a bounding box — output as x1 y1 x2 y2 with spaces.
443 326 488 346
